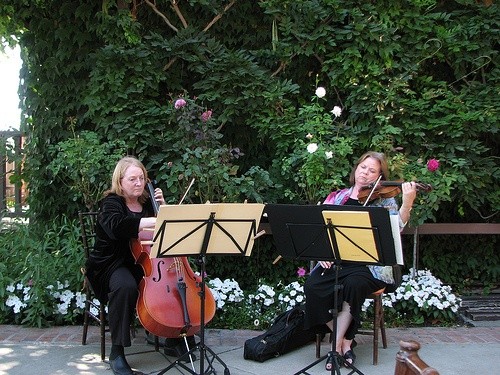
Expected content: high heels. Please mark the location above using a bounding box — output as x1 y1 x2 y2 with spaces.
325 339 357 371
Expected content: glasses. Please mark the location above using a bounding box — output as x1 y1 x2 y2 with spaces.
125 177 147 186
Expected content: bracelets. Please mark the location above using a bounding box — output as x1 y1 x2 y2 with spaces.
402 206 412 212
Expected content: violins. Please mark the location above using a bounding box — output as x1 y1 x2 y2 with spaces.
357 178 432 202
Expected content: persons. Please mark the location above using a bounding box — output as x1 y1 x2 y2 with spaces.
305 152 416 371
86 156 196 375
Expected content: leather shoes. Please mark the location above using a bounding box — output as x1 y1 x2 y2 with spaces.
163 343 196 358
109 354 133 375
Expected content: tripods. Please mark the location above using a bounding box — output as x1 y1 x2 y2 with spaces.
150 203 265 375
266 204 397 375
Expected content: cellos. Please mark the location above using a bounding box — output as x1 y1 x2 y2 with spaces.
129 177 217 339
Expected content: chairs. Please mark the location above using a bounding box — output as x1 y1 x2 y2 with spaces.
316 287 388 365
78 209 159 360
